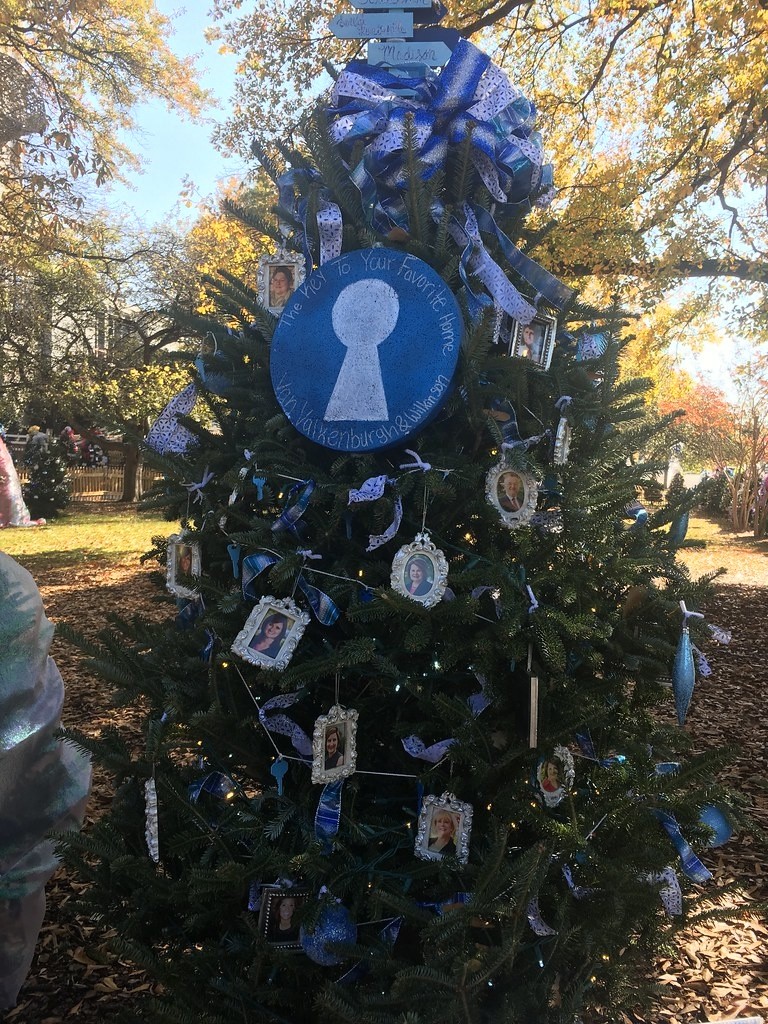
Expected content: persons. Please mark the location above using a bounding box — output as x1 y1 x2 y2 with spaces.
406 558 433 596
542 761 561 792
269 897 304 942
521 324 542 362
325 727 343 769
176 547 192 586
561 425 567 458
428 810 457 856
249 614 287 659
270 266 295 306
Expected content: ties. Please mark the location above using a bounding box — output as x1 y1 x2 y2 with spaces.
512 498 517 507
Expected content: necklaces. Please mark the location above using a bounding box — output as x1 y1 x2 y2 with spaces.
500 473 523 512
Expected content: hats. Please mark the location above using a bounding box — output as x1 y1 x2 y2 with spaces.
28 425 40 433
65 426 71 434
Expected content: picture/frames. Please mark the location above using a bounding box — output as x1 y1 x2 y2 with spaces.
254 888 314 951
229 594 311 670
255 252 306 317
483 461 538 531
311 706 359 784
536 744 575 809
412 791 474 873
506 308 558 372
165 533 202 603
390 533 449 611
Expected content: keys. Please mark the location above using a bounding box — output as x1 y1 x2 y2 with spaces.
272 759 288 795
228 544 242 579
253 474 267 500
